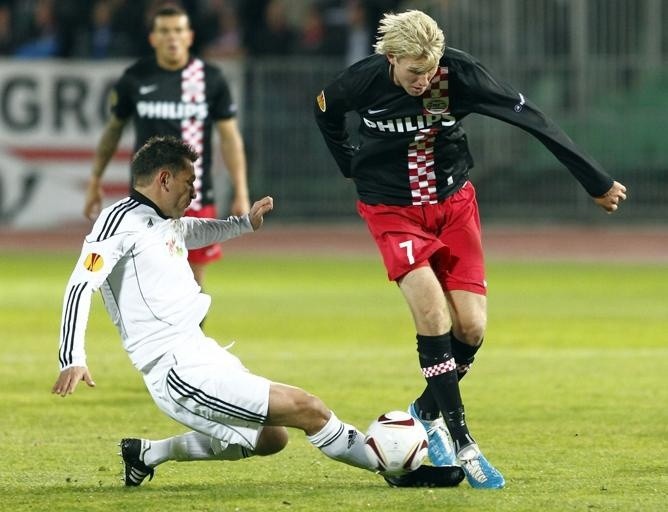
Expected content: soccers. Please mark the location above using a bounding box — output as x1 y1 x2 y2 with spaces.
363 411 428 476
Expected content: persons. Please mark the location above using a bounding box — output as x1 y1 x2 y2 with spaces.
2 1 377 61
84 3 252 329
51 138 465 488
316 10 627 489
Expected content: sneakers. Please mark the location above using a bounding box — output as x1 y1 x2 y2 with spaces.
384 403 505 490
118 437 155 486
